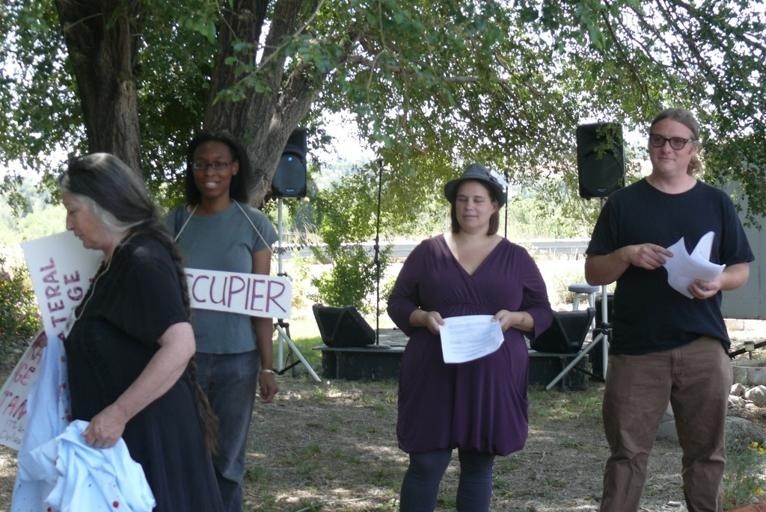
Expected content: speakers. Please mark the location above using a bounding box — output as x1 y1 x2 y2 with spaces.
576 122 625 201
270 127 306 200
592 294 613 380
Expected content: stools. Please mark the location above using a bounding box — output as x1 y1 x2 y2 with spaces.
568 283 602 332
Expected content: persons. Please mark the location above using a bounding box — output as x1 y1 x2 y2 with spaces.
587 107 753 512
387 164 553 510
31 153 226 511
160 131 279 511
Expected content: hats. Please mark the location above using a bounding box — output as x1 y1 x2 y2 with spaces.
445 163 505 208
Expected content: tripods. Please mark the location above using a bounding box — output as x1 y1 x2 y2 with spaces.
544 198 612 391
272 198 321 383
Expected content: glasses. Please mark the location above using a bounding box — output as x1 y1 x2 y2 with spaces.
189 160 232 170
649 133 693 150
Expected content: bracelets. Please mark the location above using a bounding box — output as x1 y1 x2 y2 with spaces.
260 367 273 376
